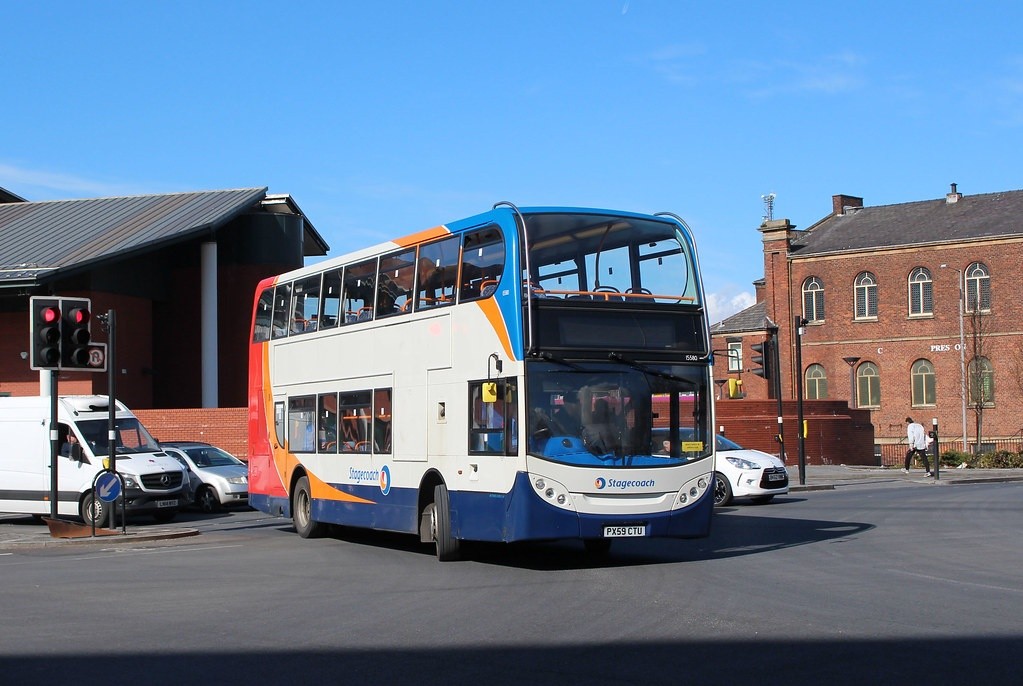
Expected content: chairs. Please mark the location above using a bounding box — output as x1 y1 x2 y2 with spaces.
288 280 655 335
327 441 372 452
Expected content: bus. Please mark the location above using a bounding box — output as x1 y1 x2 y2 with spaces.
247 201 744 561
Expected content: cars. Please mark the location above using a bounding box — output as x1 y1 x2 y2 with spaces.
134 441 248 513
647 428 791 506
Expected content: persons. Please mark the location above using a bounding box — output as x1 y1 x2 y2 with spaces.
656 441 670 454
901 417 932 477
61 434 79 462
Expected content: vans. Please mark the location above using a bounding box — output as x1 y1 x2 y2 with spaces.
0 394 194 527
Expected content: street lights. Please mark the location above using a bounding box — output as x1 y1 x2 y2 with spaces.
714 380 727 400
841 356 861 408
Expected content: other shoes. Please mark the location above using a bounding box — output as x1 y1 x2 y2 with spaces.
923 472 931 478
900 468 909 474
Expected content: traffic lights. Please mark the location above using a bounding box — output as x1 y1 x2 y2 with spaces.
803 419 808 439
929 430 935 438
33 299 91 369
751 343 769 379
774 434 780 441
